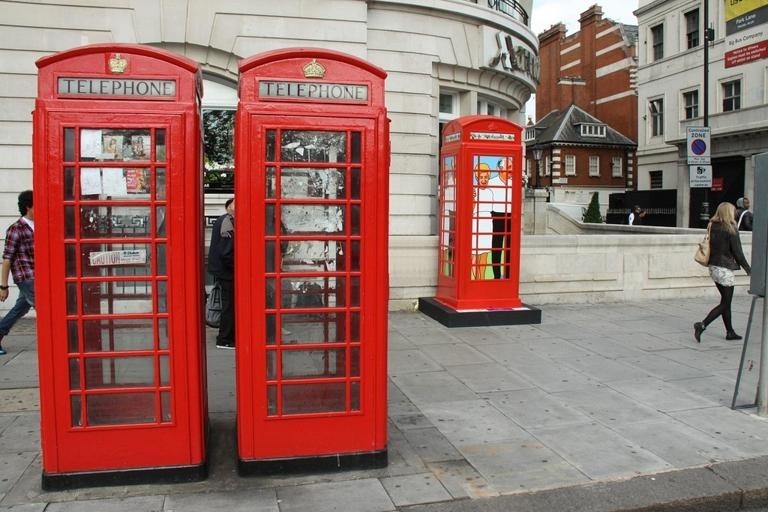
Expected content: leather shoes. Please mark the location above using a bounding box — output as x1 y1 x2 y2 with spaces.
725 328 743 341
692 320 708 345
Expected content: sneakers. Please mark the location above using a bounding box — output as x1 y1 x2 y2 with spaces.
0 340 6 354
215 340 236 350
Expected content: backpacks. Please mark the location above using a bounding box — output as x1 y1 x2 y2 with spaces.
205 274 225 329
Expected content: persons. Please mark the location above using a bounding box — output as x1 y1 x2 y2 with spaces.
134 137 146 157
694 202 751 342
208 198 235 349
0 190 35 354
280 221 291 335
107 139 119 158
629 205 646 225
441 159 512 280
735 197 753 230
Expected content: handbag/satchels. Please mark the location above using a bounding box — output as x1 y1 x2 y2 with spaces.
693 218 714 267
295 280 325 308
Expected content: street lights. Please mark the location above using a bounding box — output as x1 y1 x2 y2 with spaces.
532 144 545 189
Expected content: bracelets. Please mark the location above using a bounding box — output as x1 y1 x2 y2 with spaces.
0 286 9 290
